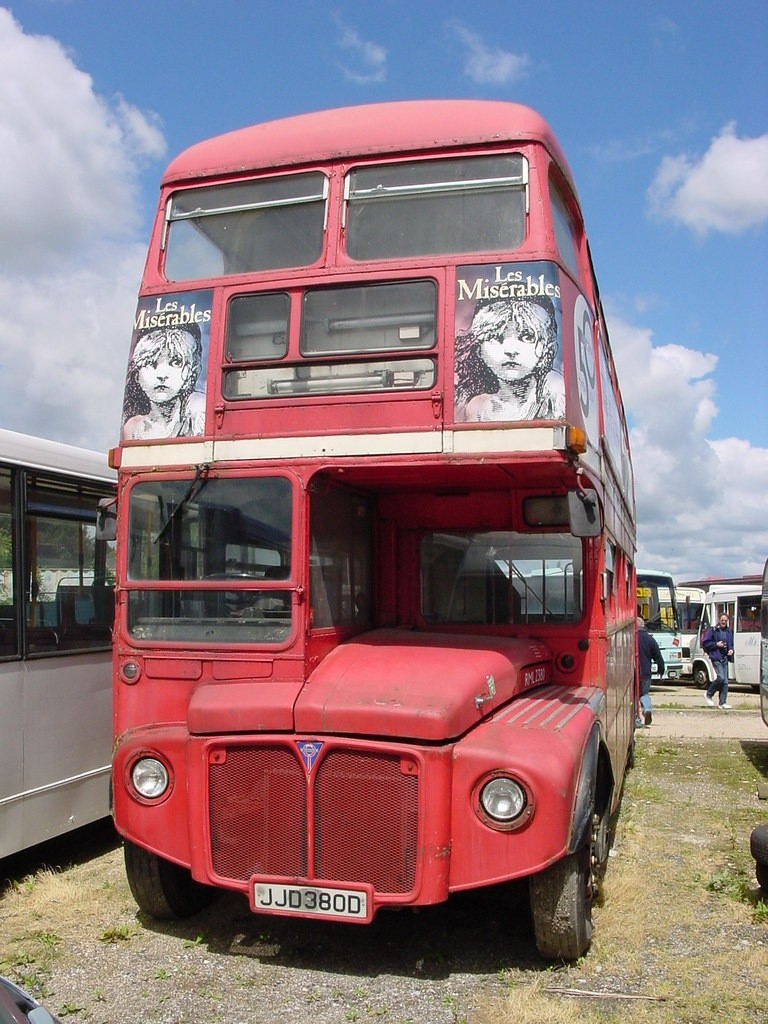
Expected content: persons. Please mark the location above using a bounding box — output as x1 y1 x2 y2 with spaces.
702 613 735 709
635 617 665 728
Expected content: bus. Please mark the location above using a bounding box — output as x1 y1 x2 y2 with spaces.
642 556 768 728
0 426 290 857
96 101 644 967
502 567 684 681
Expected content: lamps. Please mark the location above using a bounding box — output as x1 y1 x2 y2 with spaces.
323 312 434 332
268 369 394 394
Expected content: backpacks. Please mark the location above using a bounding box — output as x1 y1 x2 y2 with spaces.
700 626 716 654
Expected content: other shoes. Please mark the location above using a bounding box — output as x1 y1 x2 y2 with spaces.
718 704 731 709
644 710 652 725
704 691 712 704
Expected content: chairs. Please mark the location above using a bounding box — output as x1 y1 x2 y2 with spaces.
0 575 116 656
263 565 343 625
684 619 760 631
